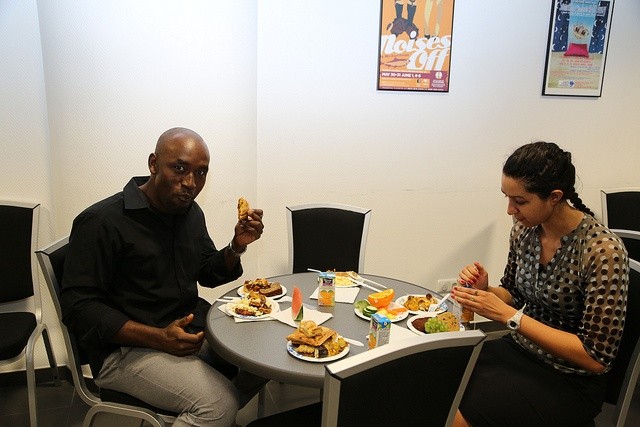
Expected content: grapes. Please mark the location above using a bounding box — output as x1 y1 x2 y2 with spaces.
425 317 449 334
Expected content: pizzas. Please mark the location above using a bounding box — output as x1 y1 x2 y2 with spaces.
238 197 250 221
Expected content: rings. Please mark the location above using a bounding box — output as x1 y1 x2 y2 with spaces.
470 295 476 301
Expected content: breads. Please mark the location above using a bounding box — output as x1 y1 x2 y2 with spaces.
438 312 460 332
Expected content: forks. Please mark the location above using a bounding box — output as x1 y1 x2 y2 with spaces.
428 292 451 312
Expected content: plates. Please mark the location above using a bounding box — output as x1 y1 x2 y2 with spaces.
395 294 449 315
286 336 350 363
237 282 288 300
318 275 364 288
406 313 466 335
225 298 279 319
354 301 410 323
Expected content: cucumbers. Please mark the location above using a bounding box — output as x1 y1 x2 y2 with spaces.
362 305 378 317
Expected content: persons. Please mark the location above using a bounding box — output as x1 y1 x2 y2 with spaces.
449 140 629 426
61 126 265 426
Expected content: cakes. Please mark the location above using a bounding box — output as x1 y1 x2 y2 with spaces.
243 278 283 296
334 271 356 287
232 295 272 316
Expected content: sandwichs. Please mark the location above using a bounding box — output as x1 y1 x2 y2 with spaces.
295 339 348 359
287 321 335 346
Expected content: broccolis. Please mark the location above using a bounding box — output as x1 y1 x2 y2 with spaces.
355 300 369 312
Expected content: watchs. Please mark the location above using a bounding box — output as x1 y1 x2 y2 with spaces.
227 239 248 257
507 309 524 330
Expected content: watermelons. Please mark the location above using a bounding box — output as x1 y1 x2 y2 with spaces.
291 287 304 321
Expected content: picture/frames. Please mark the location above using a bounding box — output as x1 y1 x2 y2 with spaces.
540 0 616 98
376 0 455 93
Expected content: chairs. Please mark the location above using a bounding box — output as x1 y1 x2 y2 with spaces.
242 326 487 427
607 228 640 266
568 257 639 426
0 199 59 426
31 233 182 427
600 186 640 231
285 200 372 277
